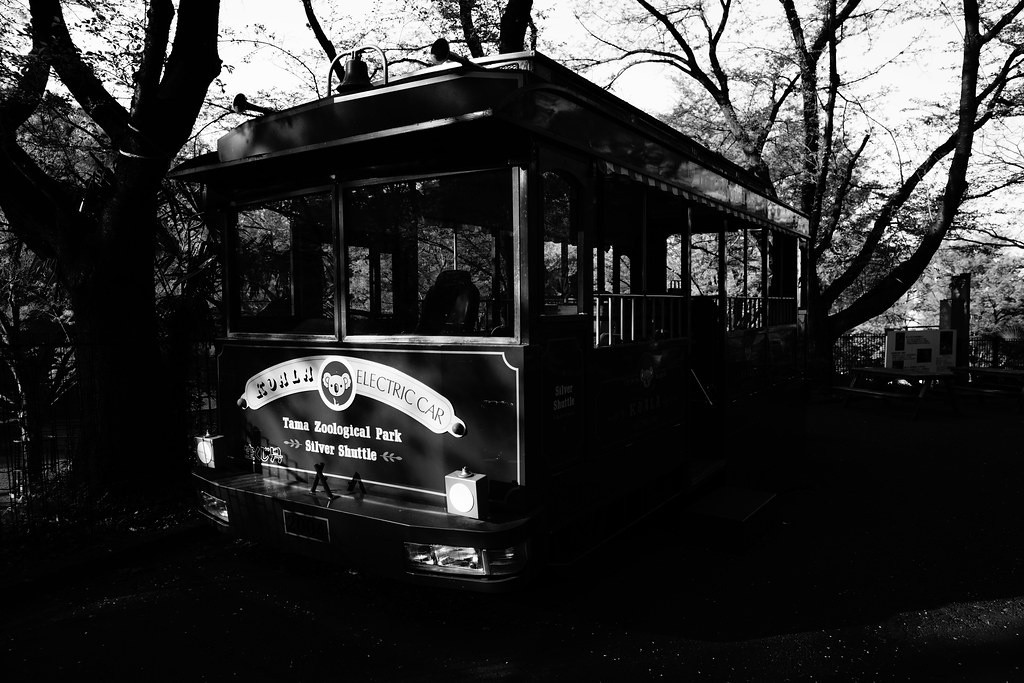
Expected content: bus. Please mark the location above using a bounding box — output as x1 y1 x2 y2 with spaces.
171 40 836 613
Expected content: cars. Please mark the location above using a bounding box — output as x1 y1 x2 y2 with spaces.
835 366 945 418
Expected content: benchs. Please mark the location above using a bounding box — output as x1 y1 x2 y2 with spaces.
828 383 1024 421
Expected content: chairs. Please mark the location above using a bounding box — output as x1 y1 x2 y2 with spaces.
415 271 481 336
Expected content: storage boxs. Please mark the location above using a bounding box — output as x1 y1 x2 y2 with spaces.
885 330 956 372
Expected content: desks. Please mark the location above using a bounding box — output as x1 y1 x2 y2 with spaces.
836 367 952 420
947 366 1024 418
